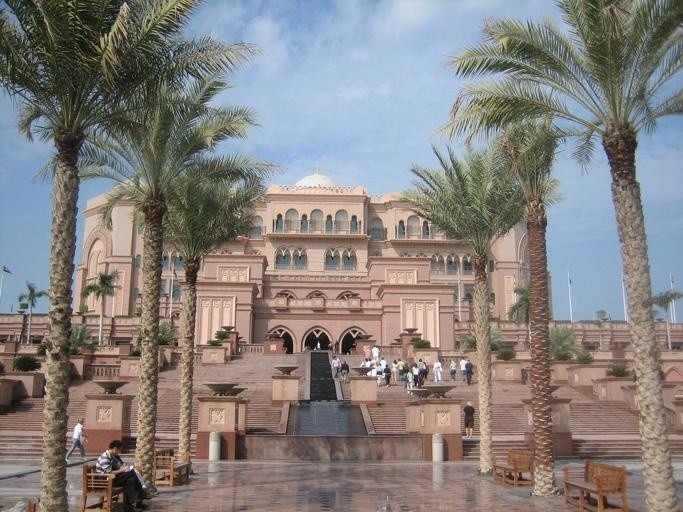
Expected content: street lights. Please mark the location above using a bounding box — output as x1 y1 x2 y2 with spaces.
162 247 182 324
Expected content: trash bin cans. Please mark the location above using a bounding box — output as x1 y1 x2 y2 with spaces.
209 431 221 461
432 433 444 462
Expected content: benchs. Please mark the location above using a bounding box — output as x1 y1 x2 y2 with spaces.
80 463 133 511
487 449 536 488
152 448 191 486
558 459 634 511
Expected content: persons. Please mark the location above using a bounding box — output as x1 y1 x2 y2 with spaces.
65 417 150 512
360 343 474 394
305 340 349 385
463 401 475 437
